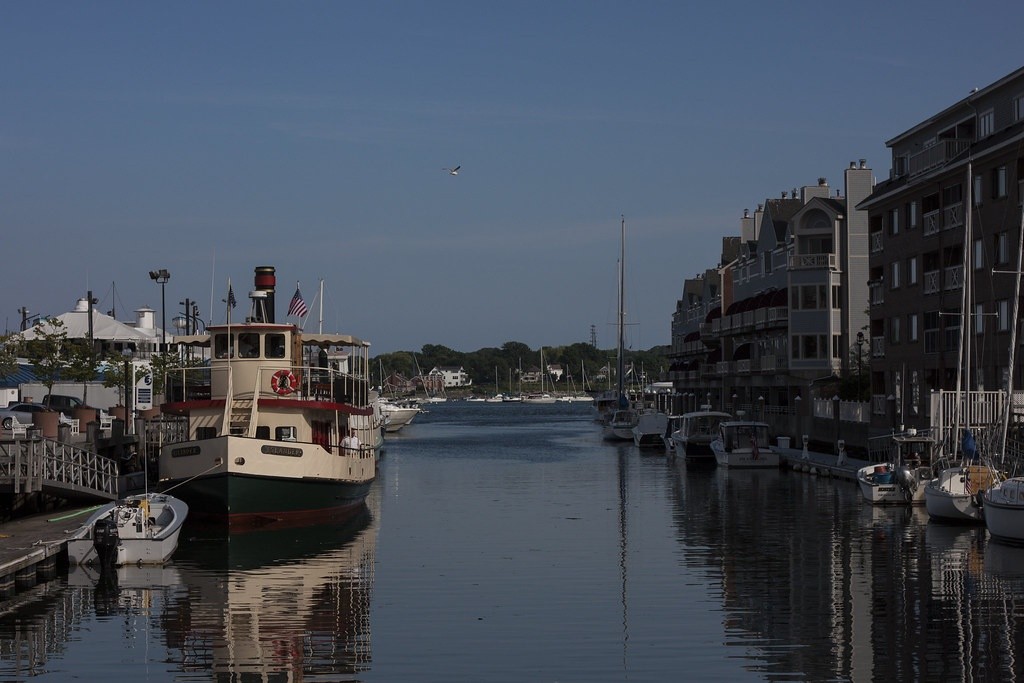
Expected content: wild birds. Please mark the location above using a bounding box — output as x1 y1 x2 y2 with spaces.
441 166 461 175
969 87 979 94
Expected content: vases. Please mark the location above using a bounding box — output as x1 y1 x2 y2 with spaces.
139 410 155 423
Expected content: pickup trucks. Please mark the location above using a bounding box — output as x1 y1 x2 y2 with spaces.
7 394 105 418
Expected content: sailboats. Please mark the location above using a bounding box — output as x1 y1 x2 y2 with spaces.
924 159 1009 526
983 209 1024 543
465 215 643 442
371 348 447 434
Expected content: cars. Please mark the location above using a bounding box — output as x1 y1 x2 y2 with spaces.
0 403 71 430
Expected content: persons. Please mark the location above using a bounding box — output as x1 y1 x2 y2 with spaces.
340 428 362 455
911 452 921 467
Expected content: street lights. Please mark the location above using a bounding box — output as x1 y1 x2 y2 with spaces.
86 289 99 365
17 306 29 330
148 268 172 397
179 297 196 335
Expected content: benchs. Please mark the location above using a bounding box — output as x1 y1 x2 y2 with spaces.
315 383 334 401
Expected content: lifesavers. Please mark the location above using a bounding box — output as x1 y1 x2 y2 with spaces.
271 369 297 394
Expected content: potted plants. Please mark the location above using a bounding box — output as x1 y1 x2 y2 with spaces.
58 330 104 431
102 350 138 430
153 367 165 407
22 312 68 437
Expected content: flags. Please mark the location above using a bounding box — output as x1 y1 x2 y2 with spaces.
287 288 308 316
229 285 237 309
751 433 759 460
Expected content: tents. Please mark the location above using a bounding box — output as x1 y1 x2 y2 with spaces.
3 298 149 340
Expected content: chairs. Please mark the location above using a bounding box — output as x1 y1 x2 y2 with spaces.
99 409 116 429
60 412 79 436
12 416 34 439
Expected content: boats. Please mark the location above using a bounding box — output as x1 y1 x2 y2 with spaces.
710 417 782 470
856 362 937 505
63 429 189 567
671 410 744 461
154 265 377 568
630 411 671 449
